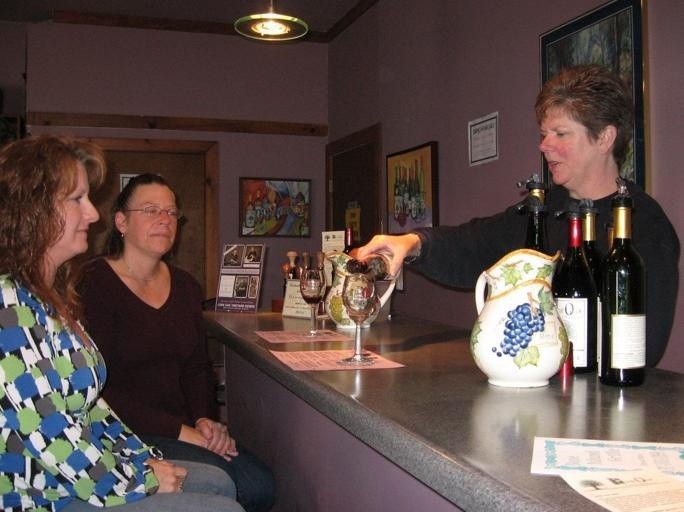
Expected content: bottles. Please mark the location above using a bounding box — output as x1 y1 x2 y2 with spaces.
391 154 427 225
282 251 325 309
559 386 654 444
344 253 389 281
524 182 648 388
245 188 263 229
342 227 355 254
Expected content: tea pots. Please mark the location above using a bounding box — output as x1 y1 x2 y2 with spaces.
469 385 561 494
469 248 570 388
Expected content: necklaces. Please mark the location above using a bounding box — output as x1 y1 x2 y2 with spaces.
123 258 158 281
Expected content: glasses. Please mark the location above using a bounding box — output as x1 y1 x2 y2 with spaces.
121 206 184 221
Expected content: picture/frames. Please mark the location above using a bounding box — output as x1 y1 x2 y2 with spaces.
386 141 439 235
538 0 651 196
238 177 312 237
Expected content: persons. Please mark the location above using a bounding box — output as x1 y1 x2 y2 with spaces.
75 172 277 511
247 246 257 262
230 250 238 262
0 132 246 511
356 64 681 367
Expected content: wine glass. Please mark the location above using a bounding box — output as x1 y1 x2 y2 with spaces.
341 279 379 367
299 269 327 338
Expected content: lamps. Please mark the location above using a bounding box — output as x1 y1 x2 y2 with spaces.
234 0 309 42
